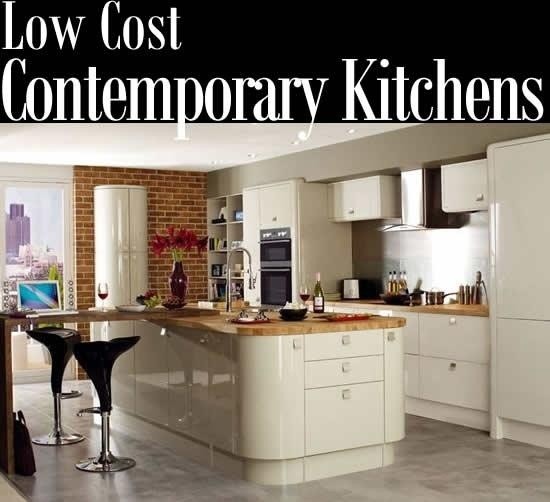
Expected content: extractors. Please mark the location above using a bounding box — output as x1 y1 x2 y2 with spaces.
377 167 461 232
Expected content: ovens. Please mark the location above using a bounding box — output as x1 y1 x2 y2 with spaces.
258 226 293 309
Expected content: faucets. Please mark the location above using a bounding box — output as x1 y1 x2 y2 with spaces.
226 247 256 314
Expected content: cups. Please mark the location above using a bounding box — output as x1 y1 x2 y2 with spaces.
235 263 241 271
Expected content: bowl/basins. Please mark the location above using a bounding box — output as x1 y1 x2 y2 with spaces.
278 308 307 321
138 298 163 307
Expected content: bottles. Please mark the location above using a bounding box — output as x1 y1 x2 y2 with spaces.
412 288 422 306
459 284 480 305
388 270 406 294
210 238 214 250
312 272 327 312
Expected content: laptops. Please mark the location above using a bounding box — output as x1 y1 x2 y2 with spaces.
16 280 79 316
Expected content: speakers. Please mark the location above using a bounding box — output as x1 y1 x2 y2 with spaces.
63 280 77 311
0 281 10 315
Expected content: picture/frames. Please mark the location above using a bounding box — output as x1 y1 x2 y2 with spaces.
211 263 222 277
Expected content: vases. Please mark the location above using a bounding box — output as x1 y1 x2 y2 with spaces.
168 262 189 301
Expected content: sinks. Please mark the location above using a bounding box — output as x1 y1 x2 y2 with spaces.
219 307 281 319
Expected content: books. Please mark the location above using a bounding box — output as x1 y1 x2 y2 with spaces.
209 237 223 250
214 283 225 297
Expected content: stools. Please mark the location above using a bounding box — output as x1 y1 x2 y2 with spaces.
72 335 142 473
27 326 85 447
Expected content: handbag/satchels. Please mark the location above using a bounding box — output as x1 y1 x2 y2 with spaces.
12 410 36 474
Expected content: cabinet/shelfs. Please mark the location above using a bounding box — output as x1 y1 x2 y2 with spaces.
418 313 491 413
336 306 417 399
496 317 550 428
493 138 549 321
327 174 401 226
258 182 297 230
304 326 384 458
440 158 490 213
206 193 244 302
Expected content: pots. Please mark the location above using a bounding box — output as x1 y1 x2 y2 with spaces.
377 292 425 303
425 288 458 306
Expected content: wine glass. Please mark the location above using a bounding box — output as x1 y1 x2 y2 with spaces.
299 285 310 306
98 282 109 313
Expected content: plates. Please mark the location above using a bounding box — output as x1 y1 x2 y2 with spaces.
326 314 369 321
163 304 187 310
115 305 146 314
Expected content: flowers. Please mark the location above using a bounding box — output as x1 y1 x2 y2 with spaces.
148 225 208 261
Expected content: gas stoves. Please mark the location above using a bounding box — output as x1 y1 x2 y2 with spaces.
369 300 426 308
